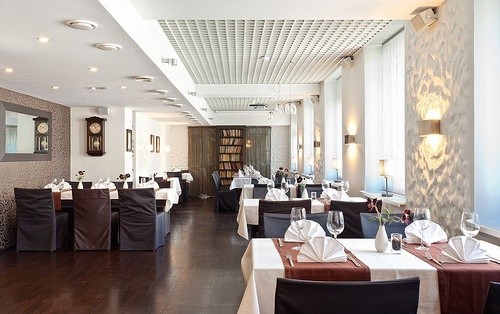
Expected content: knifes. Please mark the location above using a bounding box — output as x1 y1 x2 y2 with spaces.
277 238 283 247
346 253 361 267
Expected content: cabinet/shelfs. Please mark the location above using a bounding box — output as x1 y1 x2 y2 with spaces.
216 125 246 189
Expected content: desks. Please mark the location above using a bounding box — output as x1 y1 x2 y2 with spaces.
53 188 179 212
229 177 268 190
239 184 297 200
237 196 365 240
236 237 500 314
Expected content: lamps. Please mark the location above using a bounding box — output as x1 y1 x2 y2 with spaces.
419 119 440 137
379 159 393 197
332 158 341 182
345 135 355 145
314 141 320 148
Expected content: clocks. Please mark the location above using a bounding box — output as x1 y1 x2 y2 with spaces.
33 116 48 153
85 116 107 156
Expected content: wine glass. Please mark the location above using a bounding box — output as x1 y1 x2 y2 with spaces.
326 210 344 240
413 207 431 251
57 179 64 194
290 206 307 251
460 211 481 238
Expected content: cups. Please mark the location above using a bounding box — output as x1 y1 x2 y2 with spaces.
390 233 402 254
322 180 330 191
141 178 146 188
270 174 275 181
266 181 275 191
281 181 289 193
311 191 316 200
340 180 349 193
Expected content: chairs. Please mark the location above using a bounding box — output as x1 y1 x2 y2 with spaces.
14 169 500 314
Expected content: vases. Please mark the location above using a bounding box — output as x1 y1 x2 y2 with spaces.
280 178 285 186
303 189 308 200
375 225 388 252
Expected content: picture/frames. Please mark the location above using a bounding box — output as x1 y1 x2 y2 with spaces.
156 136 160 153
150 134 154 152
127 129 132 151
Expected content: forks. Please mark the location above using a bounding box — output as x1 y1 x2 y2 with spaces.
424 252 443 268
285 251 295 267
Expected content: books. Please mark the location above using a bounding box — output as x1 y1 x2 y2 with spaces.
220 171 236 178
220 163 241 169
220 146 242 153
221 179 232 185
220 154 239 161
220 129 242 136
221 138 242 145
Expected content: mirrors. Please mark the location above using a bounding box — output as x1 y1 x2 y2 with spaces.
0 100 52 162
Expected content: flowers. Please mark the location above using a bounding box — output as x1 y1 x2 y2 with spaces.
365 197 413 227
296 176 307 188
275 167 289 178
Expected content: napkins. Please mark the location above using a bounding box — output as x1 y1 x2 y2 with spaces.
264 187 290 201
320 187 339 199
438 234 491 266
403 218 448 247
284 218 327 243
296 235 348 264
